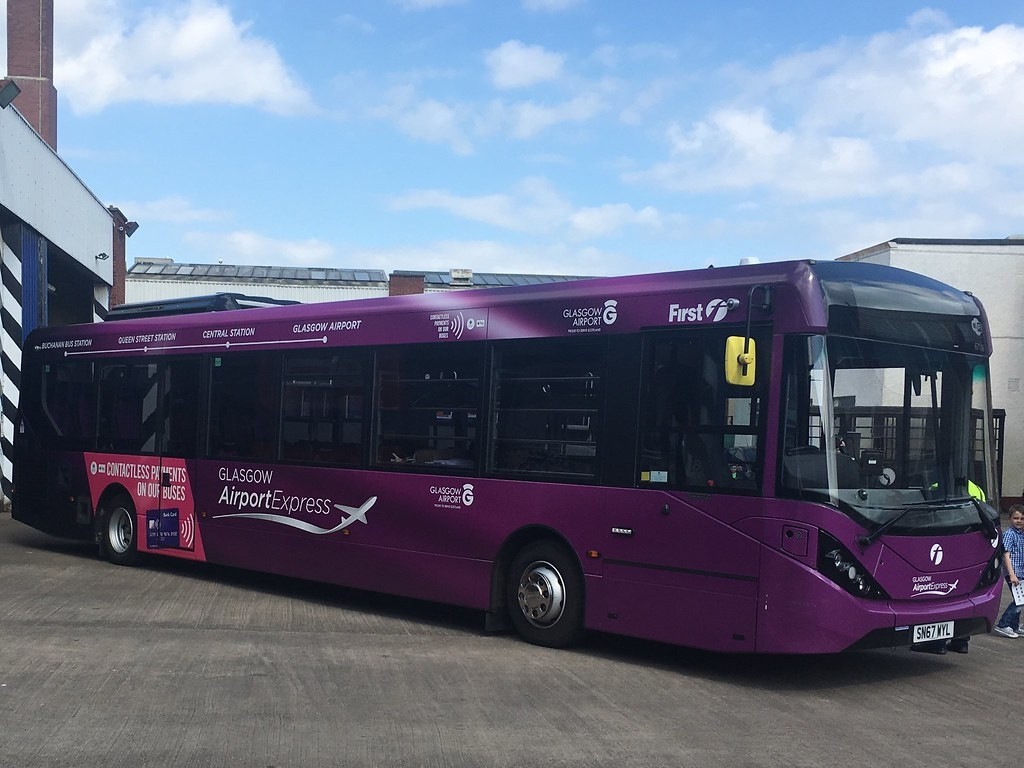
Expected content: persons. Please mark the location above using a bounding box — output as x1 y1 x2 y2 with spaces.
994 504 1024 640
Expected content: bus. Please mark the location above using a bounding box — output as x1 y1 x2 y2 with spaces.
11 259 1003 653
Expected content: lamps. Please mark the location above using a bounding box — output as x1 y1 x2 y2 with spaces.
95 252 109 260
448 267 474 286
124 221 139 237
0 80 21 109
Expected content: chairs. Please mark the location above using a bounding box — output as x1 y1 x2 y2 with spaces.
251 439 534 473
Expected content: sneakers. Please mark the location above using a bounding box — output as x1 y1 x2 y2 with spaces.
995 626 1019 638
1015 628 1024 636
